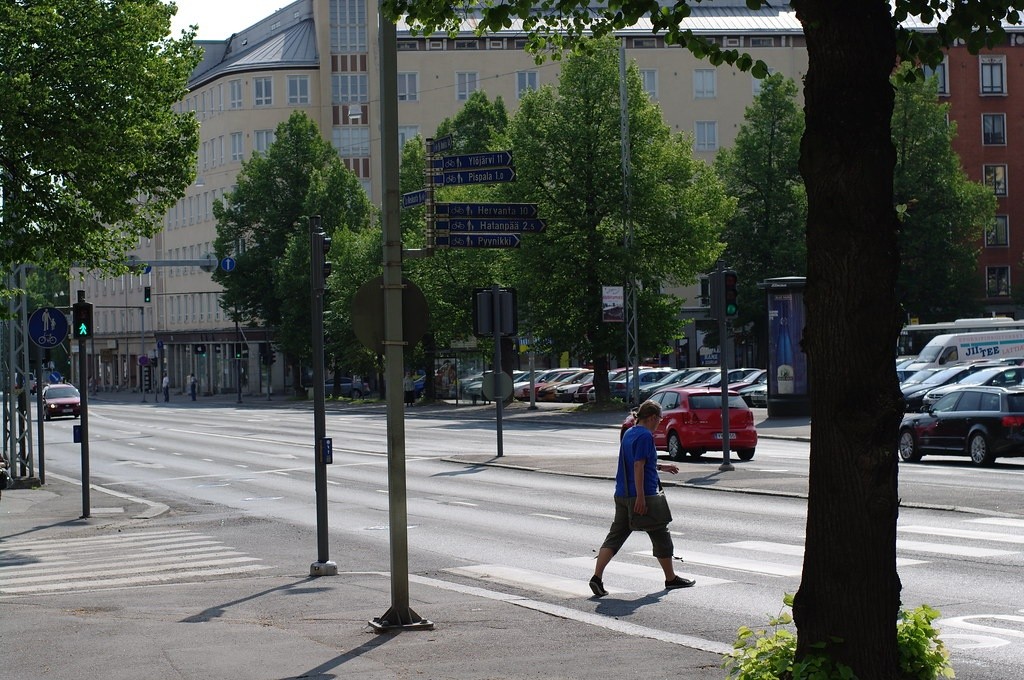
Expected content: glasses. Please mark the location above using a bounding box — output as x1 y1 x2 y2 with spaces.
658 415 663 421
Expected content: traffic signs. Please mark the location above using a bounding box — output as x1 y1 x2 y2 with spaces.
402 133 548 249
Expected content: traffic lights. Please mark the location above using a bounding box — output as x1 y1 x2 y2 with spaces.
66 359 71 365
722 270 739 319
323 237 333 277
272 351 276 363
145 287 150 302
73 303 93 339
235 343 241 359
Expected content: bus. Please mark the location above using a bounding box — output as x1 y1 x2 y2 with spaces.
897 317 1024 354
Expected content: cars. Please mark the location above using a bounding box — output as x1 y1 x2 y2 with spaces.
899 356 1024 412
453 366 767 405
305 376 370 399
620 387 758 461
412 370 425 380
896 355 919 380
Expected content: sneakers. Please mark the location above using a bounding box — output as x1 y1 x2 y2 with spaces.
665 576 696 588
589 575 608 595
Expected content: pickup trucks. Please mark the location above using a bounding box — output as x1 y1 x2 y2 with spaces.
413 369 444 389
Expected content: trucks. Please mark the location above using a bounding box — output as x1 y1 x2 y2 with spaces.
896 330 1024 383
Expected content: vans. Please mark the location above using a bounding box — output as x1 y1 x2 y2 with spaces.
7 373 36 395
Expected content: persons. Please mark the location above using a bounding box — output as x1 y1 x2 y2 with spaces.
589 400 696 596
404 372 416 407
163 373 169 402
189 374 197 401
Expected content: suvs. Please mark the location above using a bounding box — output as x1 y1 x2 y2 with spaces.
42 383 80 420
898 386 1024 468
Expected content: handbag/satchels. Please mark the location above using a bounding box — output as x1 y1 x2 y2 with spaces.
628 494 672 531
